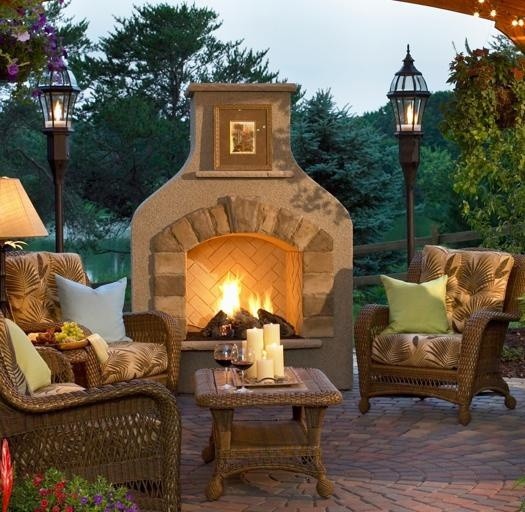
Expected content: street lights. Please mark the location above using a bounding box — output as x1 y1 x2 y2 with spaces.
35 65 81 252
386 43 431 269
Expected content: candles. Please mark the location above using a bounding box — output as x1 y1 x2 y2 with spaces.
241 323 285 385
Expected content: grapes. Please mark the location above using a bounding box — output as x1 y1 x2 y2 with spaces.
31 321 85 344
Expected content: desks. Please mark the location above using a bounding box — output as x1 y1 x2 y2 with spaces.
195 367 343 502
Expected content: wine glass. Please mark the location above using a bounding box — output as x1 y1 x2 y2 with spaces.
213 344 254 394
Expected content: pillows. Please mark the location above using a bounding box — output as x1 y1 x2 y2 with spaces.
5 318 52 395
55 273 134 343
380 274 454 335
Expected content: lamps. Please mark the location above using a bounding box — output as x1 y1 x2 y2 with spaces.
0 176 49 318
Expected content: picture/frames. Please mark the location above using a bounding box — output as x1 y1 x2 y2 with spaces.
213 104 273 171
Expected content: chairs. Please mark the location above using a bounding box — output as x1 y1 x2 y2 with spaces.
354 244 525 427
0 310 182 512
5 250 181 395
